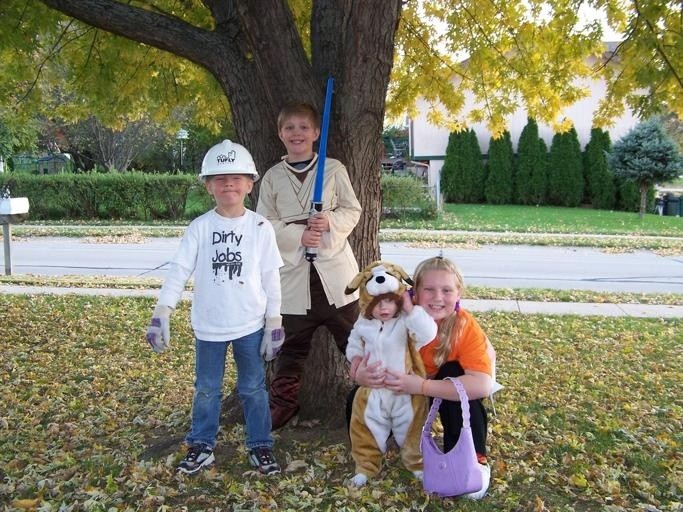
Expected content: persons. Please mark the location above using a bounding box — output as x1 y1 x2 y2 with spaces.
146 139 285 476
345 256 497 499
255 103 362 430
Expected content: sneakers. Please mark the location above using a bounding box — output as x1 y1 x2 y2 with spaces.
176 443 216 473
244 447 281 476
466 463 491 499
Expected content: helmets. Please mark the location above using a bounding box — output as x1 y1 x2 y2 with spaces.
199 139 260 182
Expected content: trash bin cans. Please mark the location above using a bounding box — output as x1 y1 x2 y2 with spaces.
663 193 683 217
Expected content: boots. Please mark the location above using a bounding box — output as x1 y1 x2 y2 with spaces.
269 374 301 431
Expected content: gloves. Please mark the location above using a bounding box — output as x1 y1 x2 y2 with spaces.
260 316 285 361
146 305 172 354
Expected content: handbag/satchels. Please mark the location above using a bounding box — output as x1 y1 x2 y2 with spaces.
421 377 482 495
